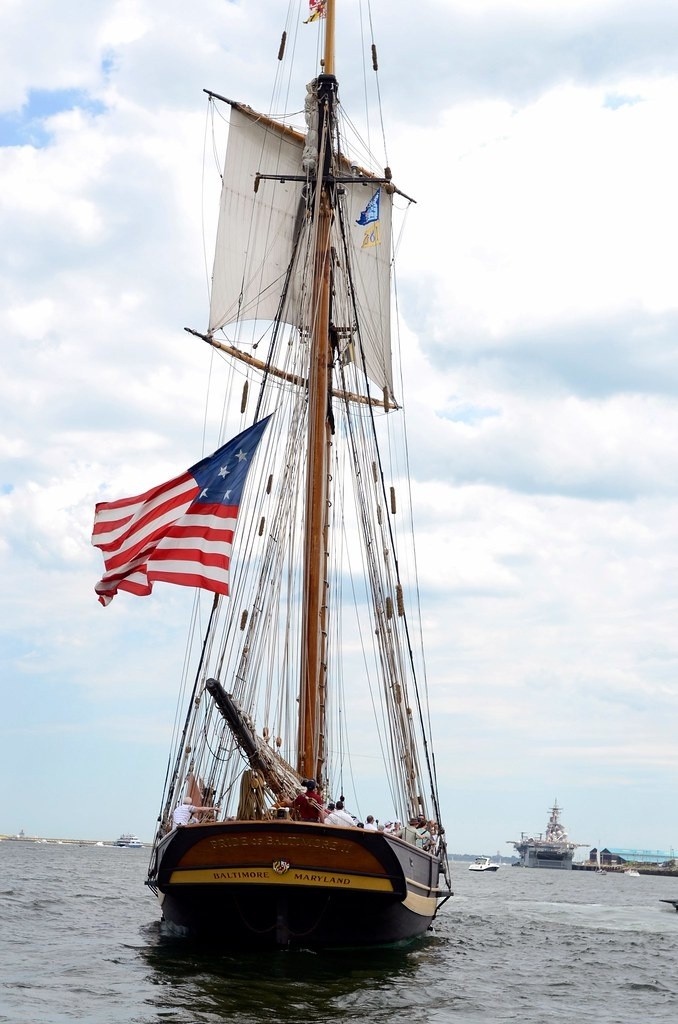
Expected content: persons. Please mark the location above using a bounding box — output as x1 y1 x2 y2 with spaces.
156 797 221 839
281 779 440 856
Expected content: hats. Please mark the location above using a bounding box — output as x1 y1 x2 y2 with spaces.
336 801 344 810
395 820 401 823
384 821 394 827
183 797 192 803
408 818 420 825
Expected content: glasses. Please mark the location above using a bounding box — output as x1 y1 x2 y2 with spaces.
431 829 435 832
314 786 319 789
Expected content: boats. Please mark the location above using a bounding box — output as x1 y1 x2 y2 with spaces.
469 857 500 871
597 869 607 875
505 797 591 870
112 834 144 849
625 868 640 877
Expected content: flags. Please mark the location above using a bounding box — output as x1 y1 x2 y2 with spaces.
339 343 353 371
90 415 270 608
361 221 380 248
356 187 379 226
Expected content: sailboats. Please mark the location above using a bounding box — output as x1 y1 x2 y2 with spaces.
147 0 455 946
